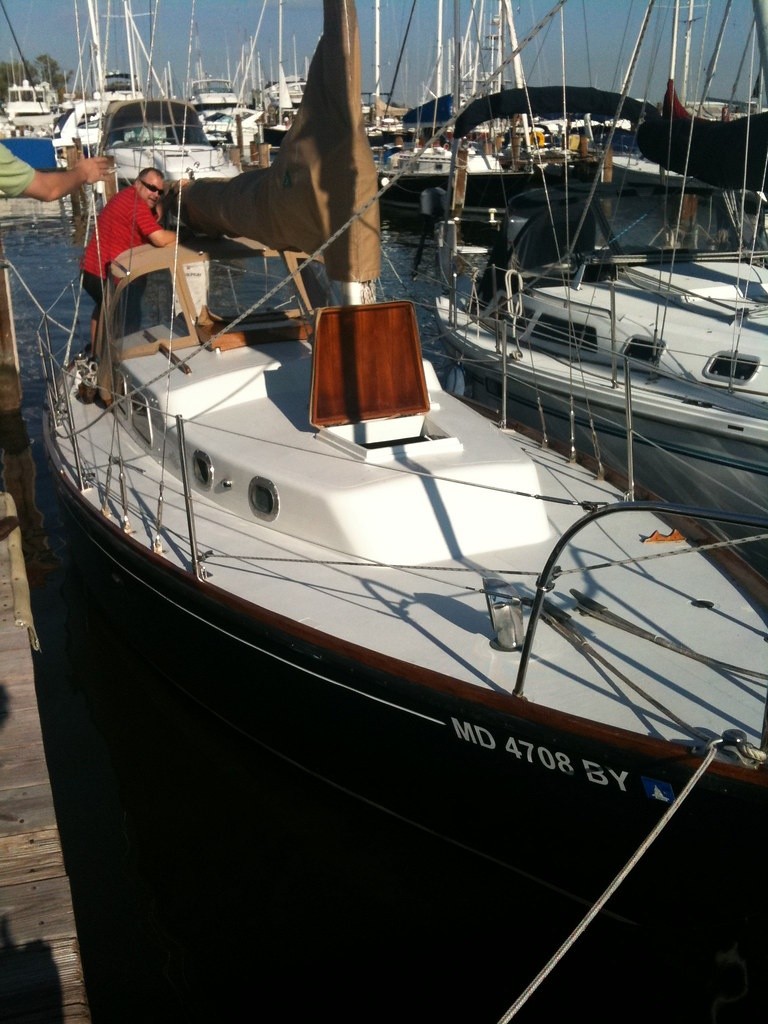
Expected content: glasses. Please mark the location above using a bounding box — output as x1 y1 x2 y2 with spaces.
138 178 165 196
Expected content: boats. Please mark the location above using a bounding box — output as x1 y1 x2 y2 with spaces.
0 0 768 1016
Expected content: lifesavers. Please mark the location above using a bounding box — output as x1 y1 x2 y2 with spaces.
530 131 545 147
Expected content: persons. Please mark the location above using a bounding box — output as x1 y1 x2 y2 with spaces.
0 141 109 203
80 167 178 411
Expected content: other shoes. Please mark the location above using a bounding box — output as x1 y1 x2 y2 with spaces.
85 357 96 371
